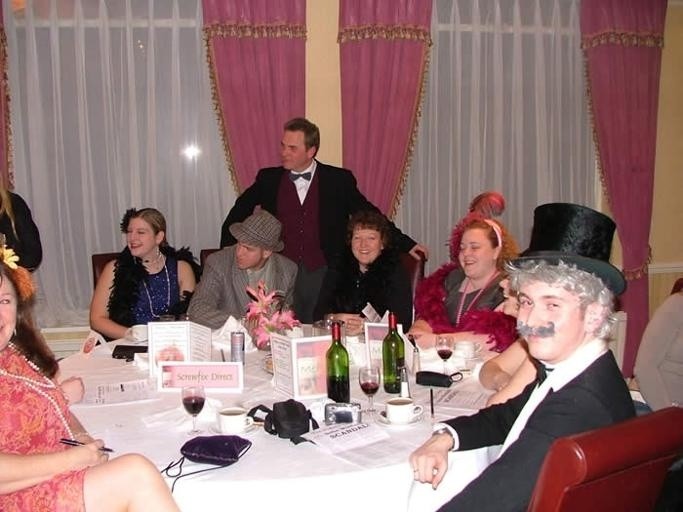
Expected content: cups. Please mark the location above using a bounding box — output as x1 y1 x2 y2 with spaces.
220 408 253 434
383 396 423 423
128 313 193 342
452 339 483 359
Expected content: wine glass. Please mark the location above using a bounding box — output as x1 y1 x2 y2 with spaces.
435 336 455 377
179 387 207 435
358 365 380 415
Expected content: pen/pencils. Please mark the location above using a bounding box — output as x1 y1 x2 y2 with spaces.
59 438 114 452
221 349 225 362
430 389 434 419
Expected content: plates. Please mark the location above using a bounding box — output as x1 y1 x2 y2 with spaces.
208 419 259 435
449 351 485 362
376 417 422 427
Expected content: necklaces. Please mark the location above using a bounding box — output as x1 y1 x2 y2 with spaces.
0 340 75 443
138 252 170 319
455 270 496 329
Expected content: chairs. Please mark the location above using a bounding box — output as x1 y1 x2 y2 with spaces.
90 250 125 347
393 251 426 327
524 406 681 512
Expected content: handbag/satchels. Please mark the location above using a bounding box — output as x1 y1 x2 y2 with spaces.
159 435 251 492
248 400 319 446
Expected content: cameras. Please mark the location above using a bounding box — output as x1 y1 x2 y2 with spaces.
325 403 361 425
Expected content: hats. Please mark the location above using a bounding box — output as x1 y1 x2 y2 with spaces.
230 210 285 252
508 202 626 296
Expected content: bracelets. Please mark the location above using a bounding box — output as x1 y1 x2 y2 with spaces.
432 427 455 452
72 432 89 439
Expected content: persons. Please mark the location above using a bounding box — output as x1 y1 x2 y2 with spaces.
408 261 635 512
216 118 429 325
635 290 683 410
0 245 185 511
0 190 42 272
403 191 520 361
311 209 413 336
86 208 197 343
478 272 540 409
183 210 298 330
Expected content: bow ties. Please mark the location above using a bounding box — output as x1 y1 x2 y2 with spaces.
288 171 313 182
537 363 548 388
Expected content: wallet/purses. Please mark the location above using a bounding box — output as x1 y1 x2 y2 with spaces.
416 371 462 387
113 345 146 363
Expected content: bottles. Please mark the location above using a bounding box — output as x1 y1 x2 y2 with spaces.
323 322 350 405
380 312 404 393
397 366 411 397
